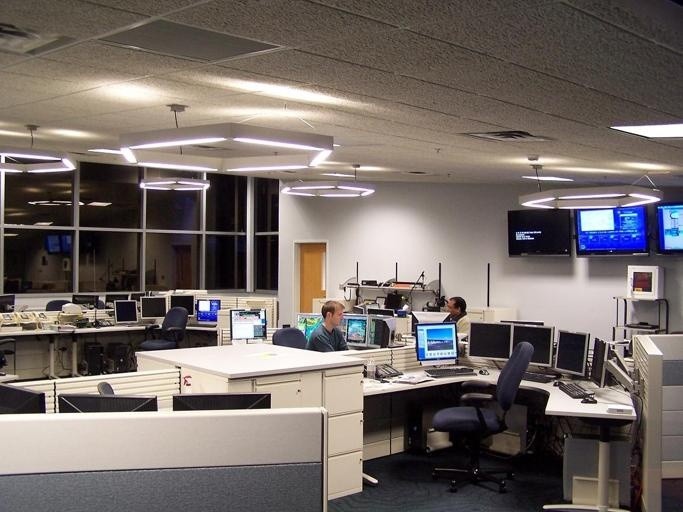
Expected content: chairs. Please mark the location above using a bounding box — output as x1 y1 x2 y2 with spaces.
431 341 534 492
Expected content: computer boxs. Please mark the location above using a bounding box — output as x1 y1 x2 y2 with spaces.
562 432 632 508
107 342 128 373
84 341 104 375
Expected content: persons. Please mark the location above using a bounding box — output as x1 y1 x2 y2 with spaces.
303 299 349 353
444 296 471 334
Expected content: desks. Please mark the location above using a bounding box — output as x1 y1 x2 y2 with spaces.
134 341 364 500
0 309 216 380
353 365 634 512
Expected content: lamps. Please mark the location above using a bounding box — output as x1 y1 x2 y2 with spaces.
140 177 210 192
0 149 76 175
116 123 335 175
30 200 112 207
519 186 663 211
282 183 373 199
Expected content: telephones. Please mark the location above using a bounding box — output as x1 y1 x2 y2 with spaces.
96 318 114 327
364 363 403 383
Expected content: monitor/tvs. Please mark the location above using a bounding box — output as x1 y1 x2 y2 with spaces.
411 311 452 337
140 296 168 319
169 294 195 317
0 294 16 313
230 308 267 344
113 300 138 323
352 306 364 315
337 316 368 347
507 209 575 259
573 205 650 258
655 201 683 255
57 392 158 413
554 330 591 377
414 321 459 361
196 299 221 321
71 294 99 309
131 292 145 301
0 383 46 413
375 321 390 348
468 321 512 362
367 308 395 318
512 324 555 368
105 294 129 309
589 337 613 389
172 392 271 410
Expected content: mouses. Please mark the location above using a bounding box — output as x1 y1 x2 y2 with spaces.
479 368 489 375
553 381 565 386
581 397 598 403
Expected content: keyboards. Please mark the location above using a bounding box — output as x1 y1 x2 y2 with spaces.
521 372 552 384
424 368 477 377
559 382 595 399
